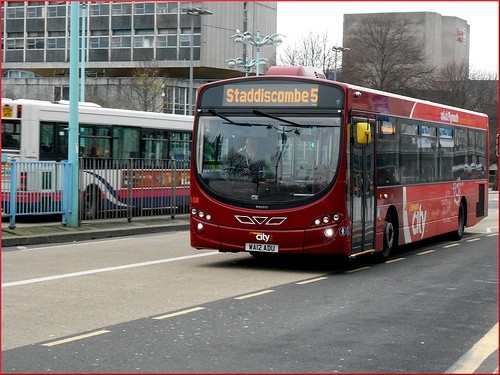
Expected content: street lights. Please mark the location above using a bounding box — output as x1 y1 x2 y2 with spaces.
183 6 214 116
224 55 270 77
331 45 351 81
230 30 287 76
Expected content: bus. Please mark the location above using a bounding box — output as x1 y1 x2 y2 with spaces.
186 65 491 266
0 97 195 220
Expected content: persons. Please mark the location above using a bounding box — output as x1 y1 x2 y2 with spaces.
459 164 471 180
478 164 485 176
468 163 483 179
353 174 373 224
228 136 265 184
